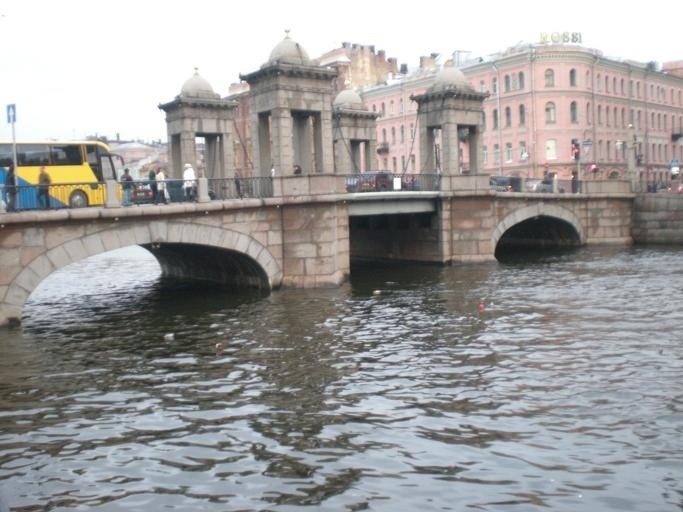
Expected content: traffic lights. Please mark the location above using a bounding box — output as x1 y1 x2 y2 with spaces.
573 143 580 159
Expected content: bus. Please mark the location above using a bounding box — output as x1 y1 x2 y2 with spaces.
0 140 128 209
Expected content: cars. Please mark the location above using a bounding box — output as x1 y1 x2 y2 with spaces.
647 178 683 194
130 177 221 203
346 171 566 194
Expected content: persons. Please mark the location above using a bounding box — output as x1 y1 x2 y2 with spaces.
234 171 243 198
148 164 157 204
3 164 16 213
120 168 136 206
180 163 195 202
293 165 301 174
153 167 169 205
35 166 51 210
